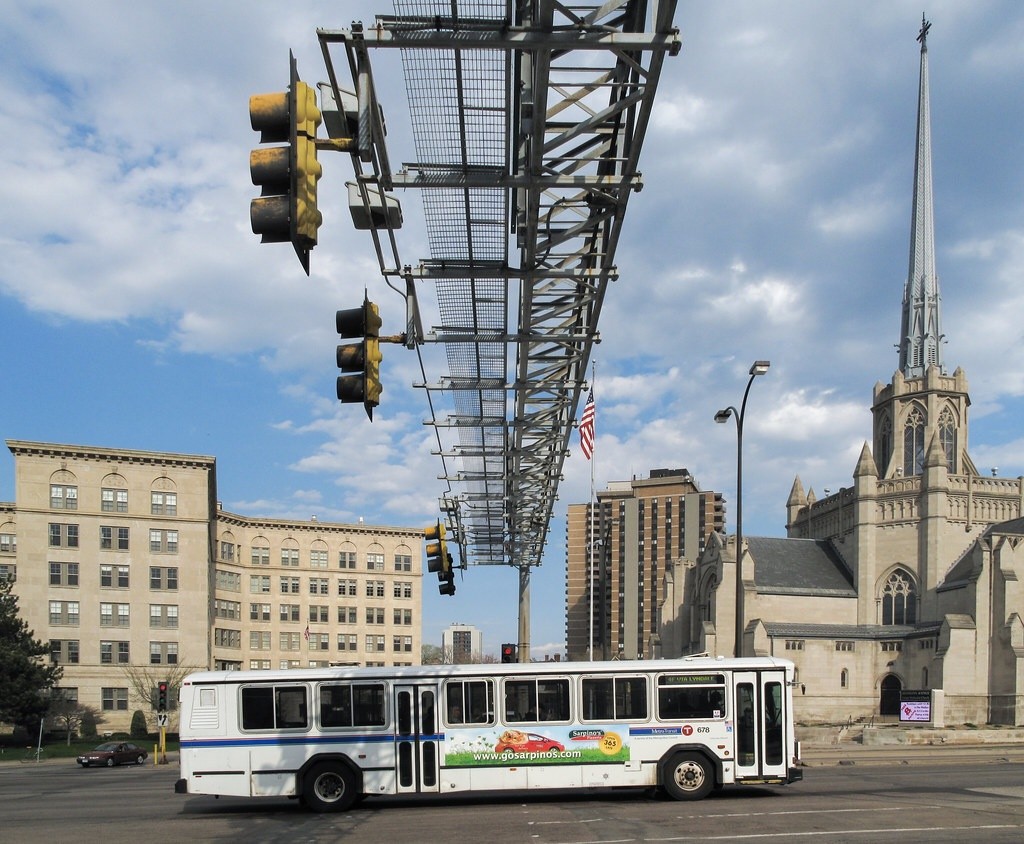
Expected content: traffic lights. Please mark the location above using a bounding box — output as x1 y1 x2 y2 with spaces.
501 643 515 663
158 681 169 713
424 517 450 574
248 48 323 277
336 283 383 421
436 559 457 598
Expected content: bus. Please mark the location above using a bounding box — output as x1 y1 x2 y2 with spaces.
175 657 811 813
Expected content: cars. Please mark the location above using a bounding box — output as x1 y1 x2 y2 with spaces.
77 741 148 767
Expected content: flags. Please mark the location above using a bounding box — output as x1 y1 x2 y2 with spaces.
580 386 598 458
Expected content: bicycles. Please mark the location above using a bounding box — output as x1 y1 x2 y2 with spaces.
19 746 48 763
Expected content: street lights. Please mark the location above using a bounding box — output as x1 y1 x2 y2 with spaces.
714 359 771 660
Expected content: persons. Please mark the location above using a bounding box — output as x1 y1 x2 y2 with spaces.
424 703 482 733
522 703 544 721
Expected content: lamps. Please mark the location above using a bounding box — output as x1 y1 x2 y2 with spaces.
991 467 999 476
824 488 830 497
896 467 904 477
